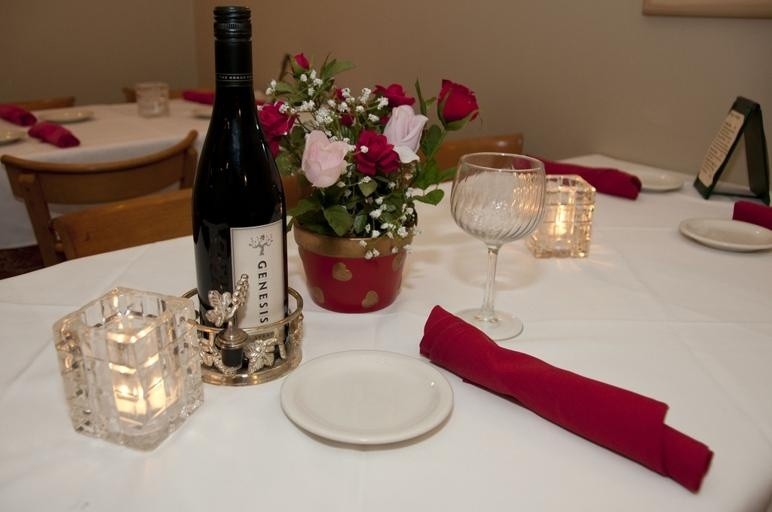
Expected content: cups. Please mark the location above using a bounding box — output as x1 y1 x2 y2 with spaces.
51 284 206 456
135 79 169 118
526 171 599 262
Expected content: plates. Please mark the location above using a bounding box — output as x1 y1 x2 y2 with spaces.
278 347 455 449
625 167 683 193
37 108 96 122
676 216 772 254
189 103 213 118
0 120 26 145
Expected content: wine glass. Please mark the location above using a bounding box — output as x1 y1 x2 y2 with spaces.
449 150 549 343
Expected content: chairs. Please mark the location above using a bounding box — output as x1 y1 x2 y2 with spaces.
435 133 528 175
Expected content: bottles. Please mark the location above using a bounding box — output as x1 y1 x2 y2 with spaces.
188 4 290 364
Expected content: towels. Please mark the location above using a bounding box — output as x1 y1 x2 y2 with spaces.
417 305 715 495
733 199 771 231
512 152 642 202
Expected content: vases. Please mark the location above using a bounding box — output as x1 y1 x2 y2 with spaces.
287 218 412 315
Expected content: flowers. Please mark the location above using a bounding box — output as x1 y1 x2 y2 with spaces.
256 52 483 258
